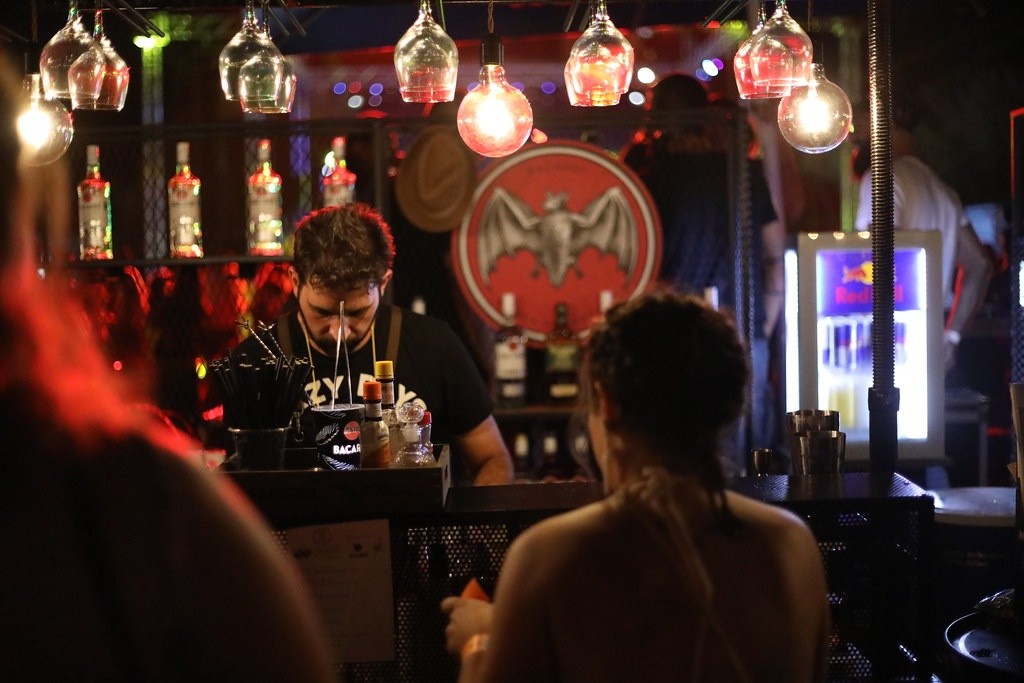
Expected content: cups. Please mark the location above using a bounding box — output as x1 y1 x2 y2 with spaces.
786 407 840 451
791 429 846 475
310 403 366 470
229 426 293 470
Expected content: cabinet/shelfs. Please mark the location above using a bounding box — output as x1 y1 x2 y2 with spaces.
61 113 755 525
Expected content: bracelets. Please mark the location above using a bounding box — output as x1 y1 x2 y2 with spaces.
946 328 963 347
461 633 489 659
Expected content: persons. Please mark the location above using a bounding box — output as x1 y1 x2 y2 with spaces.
620 72 789 477
1 61 343 682
219 202 515 487
854 77 1012 489
446 290 829 683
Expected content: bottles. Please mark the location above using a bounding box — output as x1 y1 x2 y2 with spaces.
244 139 284 257
705 285 720 314
359 381 392 469
76 144 114 262
495 292 526 407
546 303 581 407
373 360 401 469
167 142 204 259
412 296 425 315
321 137 356 208
592 292 613 332
394 402 436 467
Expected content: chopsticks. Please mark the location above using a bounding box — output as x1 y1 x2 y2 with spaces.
208 349 314 470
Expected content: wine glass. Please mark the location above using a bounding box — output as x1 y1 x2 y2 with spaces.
731 0 792 100
748 0 813 87
38 0 106 100
237 0 297 114
563 0 634 108
392 1 459 104
217 1 284 102
67 10 130 112
